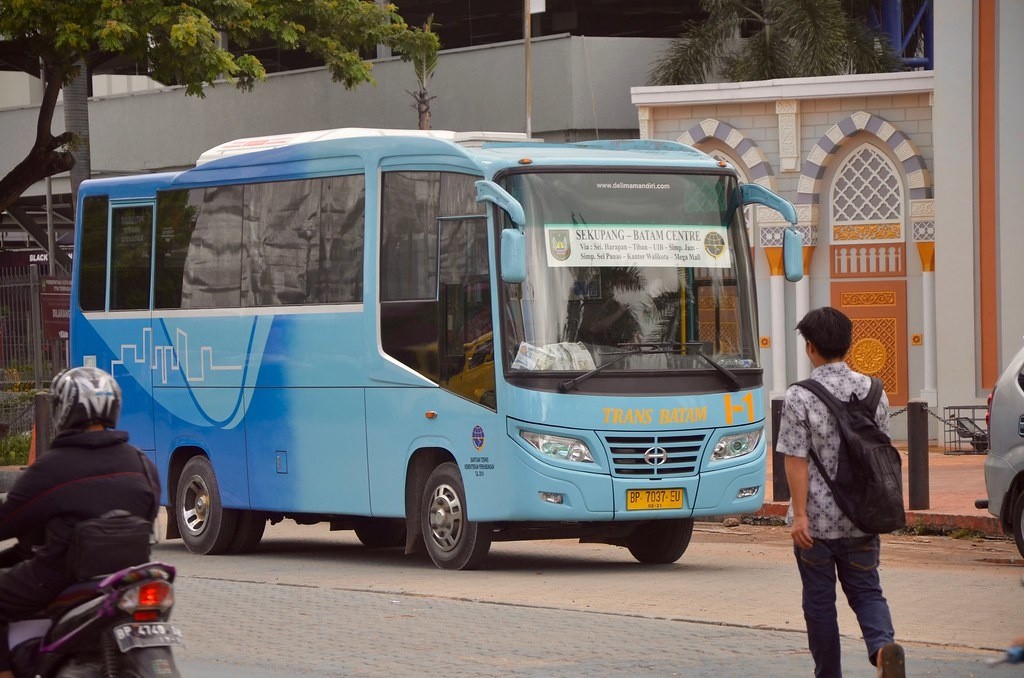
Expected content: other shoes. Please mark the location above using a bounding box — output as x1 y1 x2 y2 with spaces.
877 642 906 678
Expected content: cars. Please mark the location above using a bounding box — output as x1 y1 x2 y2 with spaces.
974 345 1024 560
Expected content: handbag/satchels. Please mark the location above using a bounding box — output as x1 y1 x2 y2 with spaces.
70 510 155 582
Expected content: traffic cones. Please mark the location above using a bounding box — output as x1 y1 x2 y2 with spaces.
19 420 37 471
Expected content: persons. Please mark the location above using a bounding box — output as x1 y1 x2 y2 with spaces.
579 270 657 348
437 301 496 409
775 306 906 678
0 366 160 678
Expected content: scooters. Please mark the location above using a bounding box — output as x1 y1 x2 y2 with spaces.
1 468 180 677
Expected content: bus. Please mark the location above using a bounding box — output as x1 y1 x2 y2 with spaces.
69 125 805 571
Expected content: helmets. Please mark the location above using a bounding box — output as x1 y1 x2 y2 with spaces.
47 366 122 431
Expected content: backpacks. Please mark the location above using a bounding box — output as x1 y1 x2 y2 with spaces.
788 374 906 534
